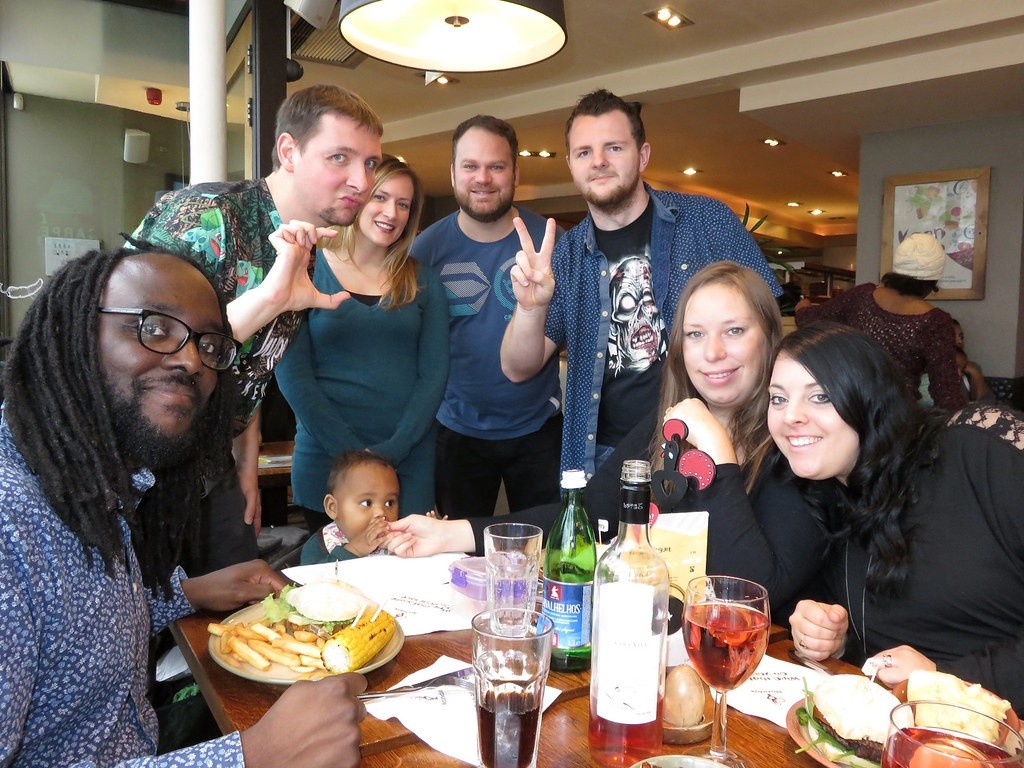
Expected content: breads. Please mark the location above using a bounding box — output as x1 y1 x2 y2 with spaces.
286 585 365 620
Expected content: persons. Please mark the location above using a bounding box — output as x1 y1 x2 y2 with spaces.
767 324 1023 721
499 90 785 488
120 83 383 568
409 115 565 518
300 453 448 564
272 155 450 535
792 234 987 409
380 260 828 611
0 245 367 768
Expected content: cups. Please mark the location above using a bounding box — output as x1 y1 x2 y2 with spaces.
471 608 555 768
483 523 543 638
880 702 1024 768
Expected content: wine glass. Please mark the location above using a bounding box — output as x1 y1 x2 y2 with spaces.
682 576 771 768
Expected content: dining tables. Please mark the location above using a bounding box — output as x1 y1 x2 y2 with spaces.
165 531 1024 768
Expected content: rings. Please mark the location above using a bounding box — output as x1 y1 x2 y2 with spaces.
884 654 891 667
799 635 806 648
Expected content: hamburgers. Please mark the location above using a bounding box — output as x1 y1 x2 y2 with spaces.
795 674 914 768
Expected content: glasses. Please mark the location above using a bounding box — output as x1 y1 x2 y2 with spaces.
94 304 241 374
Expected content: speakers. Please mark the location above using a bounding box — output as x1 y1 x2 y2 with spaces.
123 129 150 163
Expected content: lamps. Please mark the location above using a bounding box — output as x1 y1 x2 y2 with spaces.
338 0 568 73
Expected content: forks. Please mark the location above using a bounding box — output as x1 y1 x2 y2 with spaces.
356 675 475 698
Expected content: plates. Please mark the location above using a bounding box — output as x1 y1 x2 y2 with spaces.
786 694 854 768
629 754 731 768
208 603 405 685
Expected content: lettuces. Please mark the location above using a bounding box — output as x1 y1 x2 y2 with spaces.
262 583 355 638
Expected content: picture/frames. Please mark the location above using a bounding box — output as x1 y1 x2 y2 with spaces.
878 164 995 301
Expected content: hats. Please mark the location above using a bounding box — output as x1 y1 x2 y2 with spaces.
892 234 946 280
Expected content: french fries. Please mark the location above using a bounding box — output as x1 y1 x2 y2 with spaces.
208 615 325 680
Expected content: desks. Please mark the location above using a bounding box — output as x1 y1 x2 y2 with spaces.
257 440 295 476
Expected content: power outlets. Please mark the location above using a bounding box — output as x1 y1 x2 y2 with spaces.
44 237 100 276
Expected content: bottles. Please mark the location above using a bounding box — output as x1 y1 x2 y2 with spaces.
587 460 670 767
541 470 597 673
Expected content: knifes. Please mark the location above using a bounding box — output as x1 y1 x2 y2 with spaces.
361 667 474 702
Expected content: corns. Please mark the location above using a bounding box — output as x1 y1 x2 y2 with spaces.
320 612 396 673
